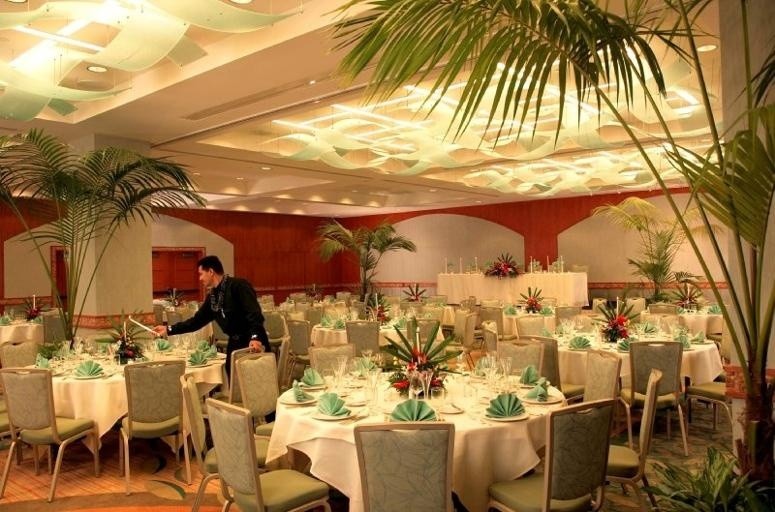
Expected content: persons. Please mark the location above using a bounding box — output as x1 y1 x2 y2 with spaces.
150 255 276 423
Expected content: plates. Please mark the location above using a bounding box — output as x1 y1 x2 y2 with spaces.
552 332 714 353
24 350 227 380
279 367 562 422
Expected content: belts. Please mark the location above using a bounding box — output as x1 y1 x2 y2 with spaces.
228 334 252 341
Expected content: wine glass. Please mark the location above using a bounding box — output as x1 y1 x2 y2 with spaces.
677 303 711 316
52 334 192 374
321 350 513 422
332 301 417 326
558 315 690 347
